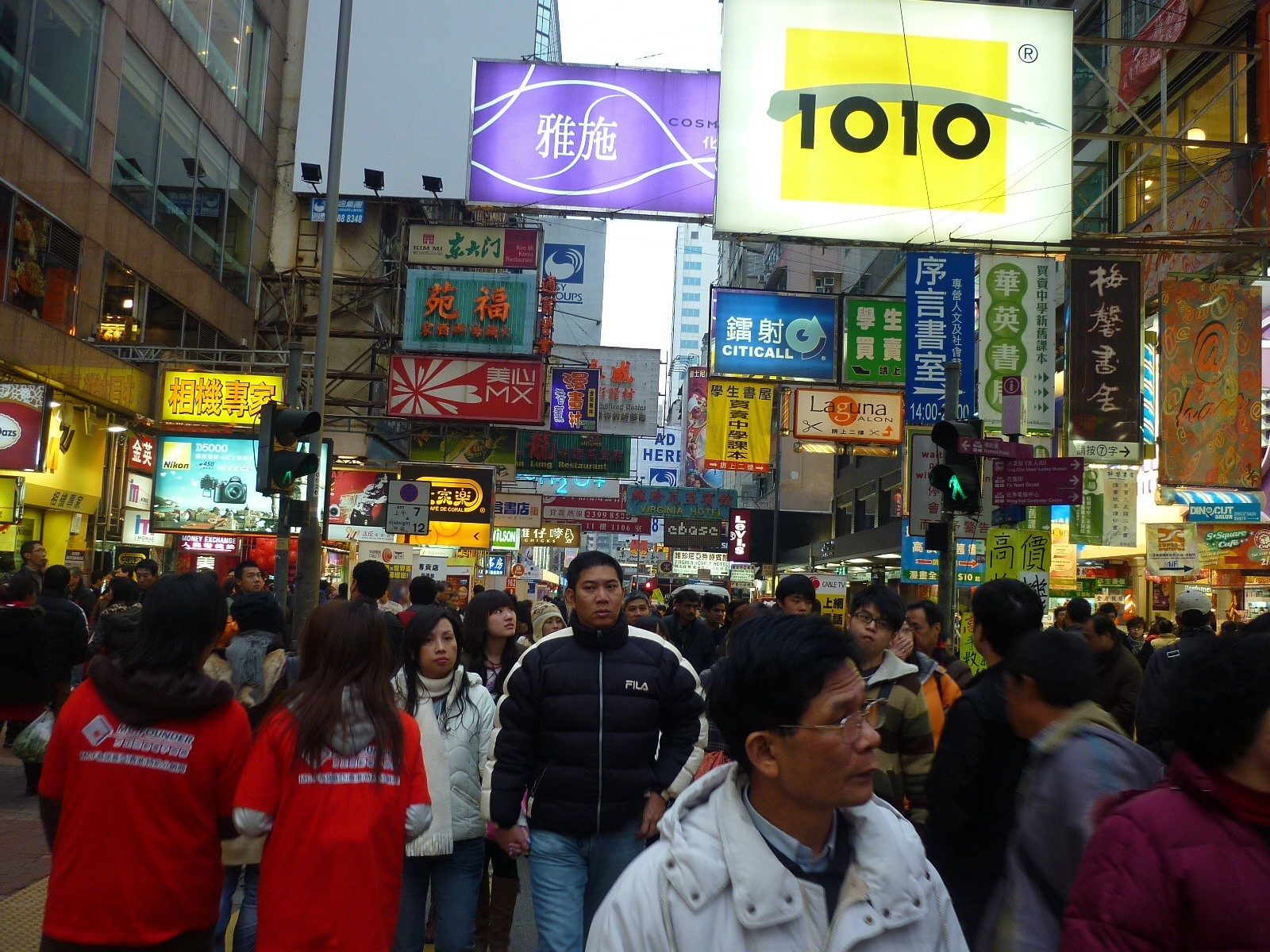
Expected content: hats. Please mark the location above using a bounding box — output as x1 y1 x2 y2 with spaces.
1173 591 1212 621
531 601 568 642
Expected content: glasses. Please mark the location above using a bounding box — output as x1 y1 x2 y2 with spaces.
851 612 893 631
762 697 890 744
28 549 45 553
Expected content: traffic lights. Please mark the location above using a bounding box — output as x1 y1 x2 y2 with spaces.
256 404 322 494
928 416 984 512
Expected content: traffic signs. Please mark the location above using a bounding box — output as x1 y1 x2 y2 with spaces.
1146 522 1201 577
958 436 1034 459
991 456 1085 506
901 516 985 587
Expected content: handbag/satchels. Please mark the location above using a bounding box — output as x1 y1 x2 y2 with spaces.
12 710 55 762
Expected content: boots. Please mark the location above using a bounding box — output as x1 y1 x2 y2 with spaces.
473 872 490 952
487 873 521 952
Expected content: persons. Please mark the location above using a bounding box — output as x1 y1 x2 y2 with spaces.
490 550 707 952
1063 612 1269 952
0 542 1270 952
586 614 971 951
230 600 433 951
39 572 252 952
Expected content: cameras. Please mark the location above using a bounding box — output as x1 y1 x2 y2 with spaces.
214 476 247 504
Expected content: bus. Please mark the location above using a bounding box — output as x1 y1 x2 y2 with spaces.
623 574 732 604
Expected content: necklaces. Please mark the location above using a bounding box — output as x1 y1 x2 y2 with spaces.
487 658 502 667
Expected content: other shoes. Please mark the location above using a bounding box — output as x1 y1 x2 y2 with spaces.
3 737 13 747
25 783 38 795
